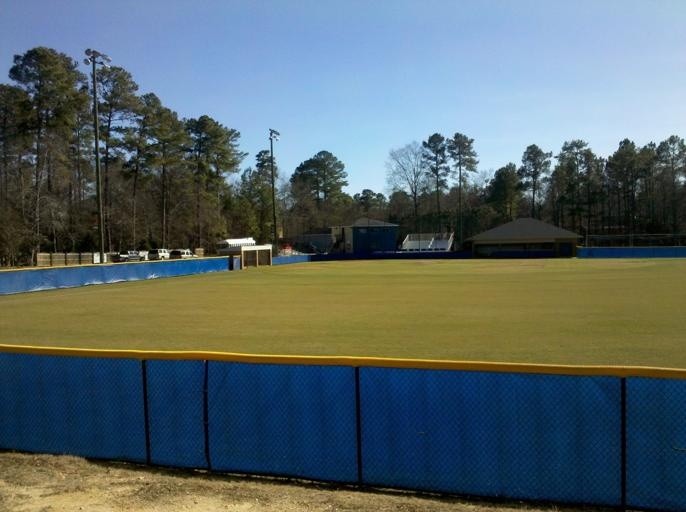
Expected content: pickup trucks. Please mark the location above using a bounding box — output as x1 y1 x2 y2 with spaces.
110 249 144 262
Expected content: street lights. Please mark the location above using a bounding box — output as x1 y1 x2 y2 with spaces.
83 45 111 264
267 127 279 243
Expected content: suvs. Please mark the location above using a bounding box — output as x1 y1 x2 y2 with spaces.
147 248 169 260
169 250 198 258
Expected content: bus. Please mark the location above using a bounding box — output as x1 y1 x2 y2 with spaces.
215 236 256 255
